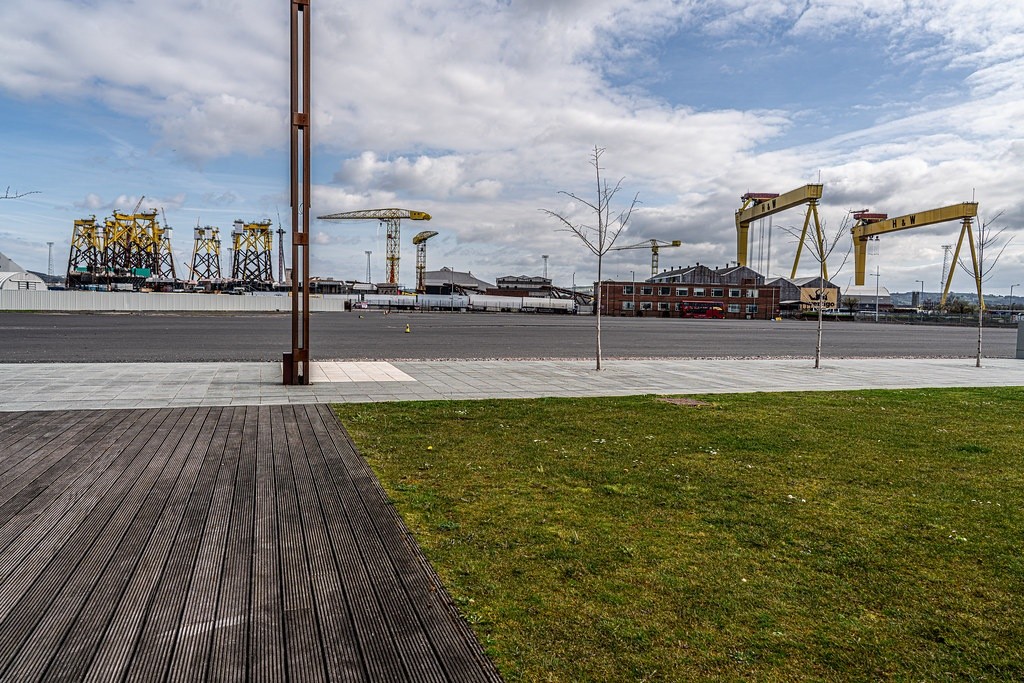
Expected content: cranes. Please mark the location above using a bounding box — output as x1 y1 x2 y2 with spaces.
316 209 439 294
602 237 682 275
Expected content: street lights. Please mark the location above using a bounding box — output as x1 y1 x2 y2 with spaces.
915 280 924 303
1009 284 1020 309
629 270 636 316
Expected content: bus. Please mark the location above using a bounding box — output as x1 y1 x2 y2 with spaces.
679 300 726 319
